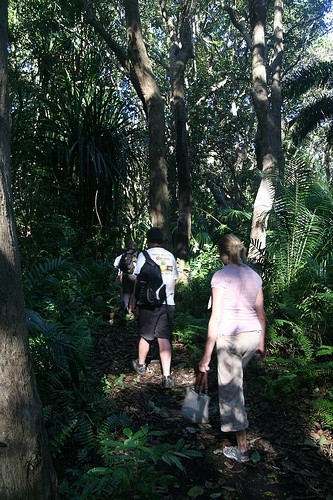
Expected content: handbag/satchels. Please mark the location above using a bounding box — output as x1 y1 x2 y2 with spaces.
180 371 210 423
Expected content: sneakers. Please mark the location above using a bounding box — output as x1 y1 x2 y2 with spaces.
130 359 145 375
222 446 249 463
161 376 172 388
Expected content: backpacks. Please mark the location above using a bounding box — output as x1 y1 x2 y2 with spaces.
118 253 137 274
135 250 165 311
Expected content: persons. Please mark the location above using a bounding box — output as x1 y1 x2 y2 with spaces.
131 227 178 389
198 234 265 463
113 240 140 316
